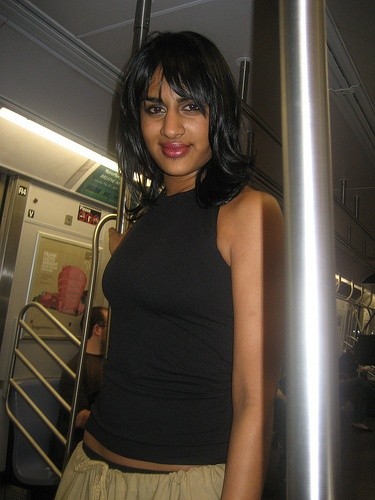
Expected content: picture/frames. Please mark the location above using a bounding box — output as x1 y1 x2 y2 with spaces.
20 231 103 342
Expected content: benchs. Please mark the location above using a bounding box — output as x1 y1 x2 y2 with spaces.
7 377 67 486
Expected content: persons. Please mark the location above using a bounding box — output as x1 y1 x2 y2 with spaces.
334 334 373 431
51 30 286 500
60 306 110 444
